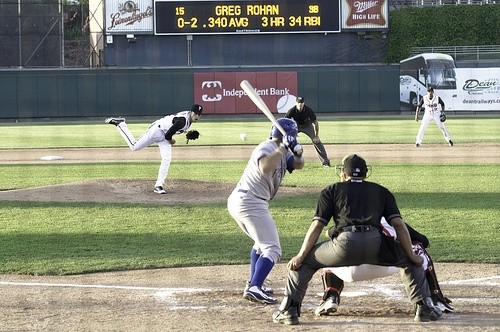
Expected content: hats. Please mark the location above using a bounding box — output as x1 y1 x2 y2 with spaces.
344 155 367 177
192 104 203 115
296 97 304 102
427 87 433 91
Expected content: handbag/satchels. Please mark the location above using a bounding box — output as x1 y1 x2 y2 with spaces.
377 230 397 264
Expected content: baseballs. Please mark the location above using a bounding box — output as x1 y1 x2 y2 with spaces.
240 133 248 141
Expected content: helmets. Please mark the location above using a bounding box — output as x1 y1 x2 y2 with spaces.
271 118 298 139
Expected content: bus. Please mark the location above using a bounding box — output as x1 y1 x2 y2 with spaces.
399 52 457 106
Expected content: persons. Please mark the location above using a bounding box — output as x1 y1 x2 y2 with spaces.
270 154 441 326
315 218 454 316
228 118 305 305
284 96 331 167
105 104 203 194
416 87 453 147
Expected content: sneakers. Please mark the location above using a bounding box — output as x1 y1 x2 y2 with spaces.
413 305 441 322
105 116 125 125
243 285 272 298
435 302 459 313
154 186 166 194
316 295 338 315
246 286 277 304
273 307 299 323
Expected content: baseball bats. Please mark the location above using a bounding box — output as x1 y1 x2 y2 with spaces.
240 79 286 136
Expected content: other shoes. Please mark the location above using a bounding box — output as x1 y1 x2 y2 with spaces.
416 143 419 147
325 163 330 167
449 140 453 146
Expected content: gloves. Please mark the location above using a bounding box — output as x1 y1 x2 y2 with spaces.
281 132 303 156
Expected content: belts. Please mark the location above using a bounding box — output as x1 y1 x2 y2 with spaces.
158 125 160 128
343 226 375 232
238 189 266 200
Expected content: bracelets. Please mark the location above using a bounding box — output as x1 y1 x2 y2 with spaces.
296 150 303 158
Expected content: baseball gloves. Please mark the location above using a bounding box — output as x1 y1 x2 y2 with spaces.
439 113 446 122
186 130 199 140
